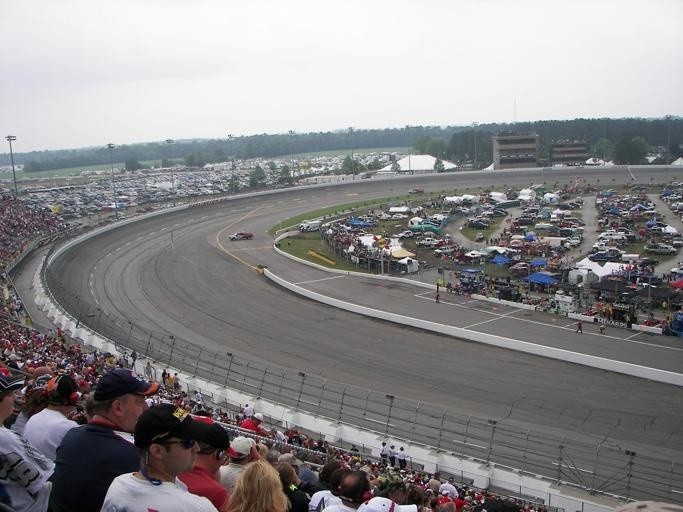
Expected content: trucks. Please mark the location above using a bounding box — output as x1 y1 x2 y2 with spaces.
299 219 320 232
585 158 602 165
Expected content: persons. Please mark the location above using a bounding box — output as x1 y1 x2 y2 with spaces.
0 189 71 511
319 202 433 274
433 181 682 335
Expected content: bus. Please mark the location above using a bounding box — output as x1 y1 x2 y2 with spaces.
661 267 683 289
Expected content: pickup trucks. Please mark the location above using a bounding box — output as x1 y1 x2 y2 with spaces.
229 231 253 241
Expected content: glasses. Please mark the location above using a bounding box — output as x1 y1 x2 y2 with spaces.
164 438 197 448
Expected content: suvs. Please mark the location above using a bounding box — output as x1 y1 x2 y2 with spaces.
637 274 660 288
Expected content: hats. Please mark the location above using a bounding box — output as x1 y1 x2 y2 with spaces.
228 436 257 459
380 441 474 502
254 412 263 421
278 453 303 466
92 368 160 401
0 374 25 395
16 361 25 369
356 496 417 512
8 354 22 361
132 403 209 446
46 374 78 402
200 422 230 449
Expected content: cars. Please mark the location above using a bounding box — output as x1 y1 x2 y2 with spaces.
574 162 580 166
581 161 585 165
567 163 572 166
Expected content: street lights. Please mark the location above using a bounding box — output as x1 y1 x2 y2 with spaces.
288 130 297 184
348 126 356 180
106 142 119 219
4 135 18 197
164 138 177 207
405 124 412 174
665 113 672 166
470 122 479 171
227 133 236 195
